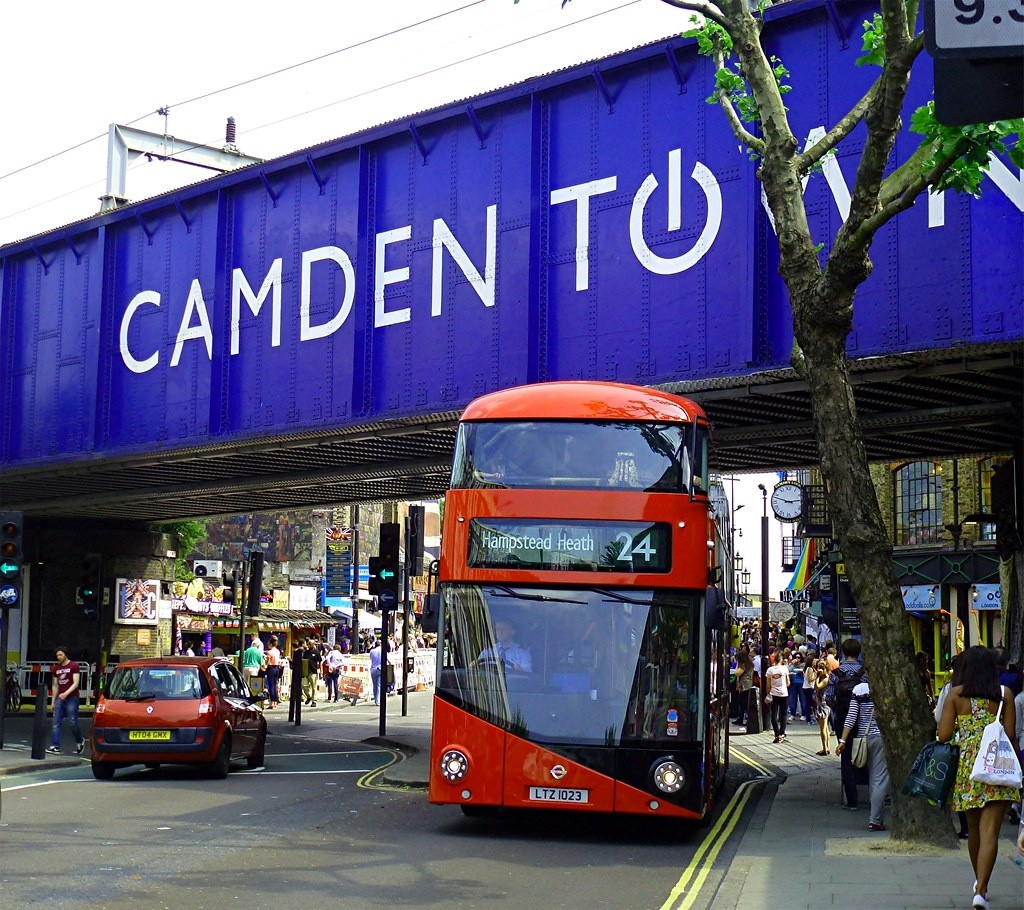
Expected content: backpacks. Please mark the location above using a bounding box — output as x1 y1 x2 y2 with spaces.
831 666 868 723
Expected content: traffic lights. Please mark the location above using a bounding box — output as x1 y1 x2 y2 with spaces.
223 568 238 606
79 552 99 622
0 510 24 609
368 555 399 610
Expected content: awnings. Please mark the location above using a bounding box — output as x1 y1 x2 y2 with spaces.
785 534 814 591
211 610 335 623
336 607 390 625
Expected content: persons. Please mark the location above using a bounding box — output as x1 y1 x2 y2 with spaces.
825 638 1024 862
730 606 840 756
183 621 451 710
45 647 85 755
470 618 534 672
935 645 1024 910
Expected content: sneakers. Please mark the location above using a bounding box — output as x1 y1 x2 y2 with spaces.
45 745 61 755
76 737 87 753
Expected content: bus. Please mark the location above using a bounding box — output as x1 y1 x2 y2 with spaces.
419 380 742 842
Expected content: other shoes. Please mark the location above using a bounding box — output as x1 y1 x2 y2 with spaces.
305 697 312 704
267 704 277 709
956 830 970 840
731 719 742 726
375 703 379 706
789 715 795 720
799 715 806 721
842 803 859 811
816 750 830 755
311 702 317 706
773 736 784 744
325 699 331 702
780 734 790 741
334 699 339 703
1008 803 1019 825
973 880 990 901
868 820 885 832
972 893 992 910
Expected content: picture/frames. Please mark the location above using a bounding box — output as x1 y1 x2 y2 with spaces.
114 577 161 626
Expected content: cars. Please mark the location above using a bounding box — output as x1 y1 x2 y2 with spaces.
90 656 267 781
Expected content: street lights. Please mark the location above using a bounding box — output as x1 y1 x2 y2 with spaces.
757 483 771 732
740 568 752 623
733 550 743 626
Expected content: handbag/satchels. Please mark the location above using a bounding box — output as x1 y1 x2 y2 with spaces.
764 693 773 705
899 739 962 811
851 735 869 768
969 687 1024 790
278 664 285 678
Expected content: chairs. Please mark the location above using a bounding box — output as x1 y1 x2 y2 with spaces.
145 678 166 692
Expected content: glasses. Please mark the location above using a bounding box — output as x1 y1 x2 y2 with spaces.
794 657 801 659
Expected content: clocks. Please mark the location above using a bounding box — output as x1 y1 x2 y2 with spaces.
771 484 804 520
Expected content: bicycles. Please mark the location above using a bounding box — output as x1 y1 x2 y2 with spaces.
5 666 22 711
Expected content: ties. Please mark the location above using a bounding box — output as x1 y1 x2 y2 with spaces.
499 647 509 660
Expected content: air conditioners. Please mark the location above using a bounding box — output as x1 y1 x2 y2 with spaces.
193 560 223 578
